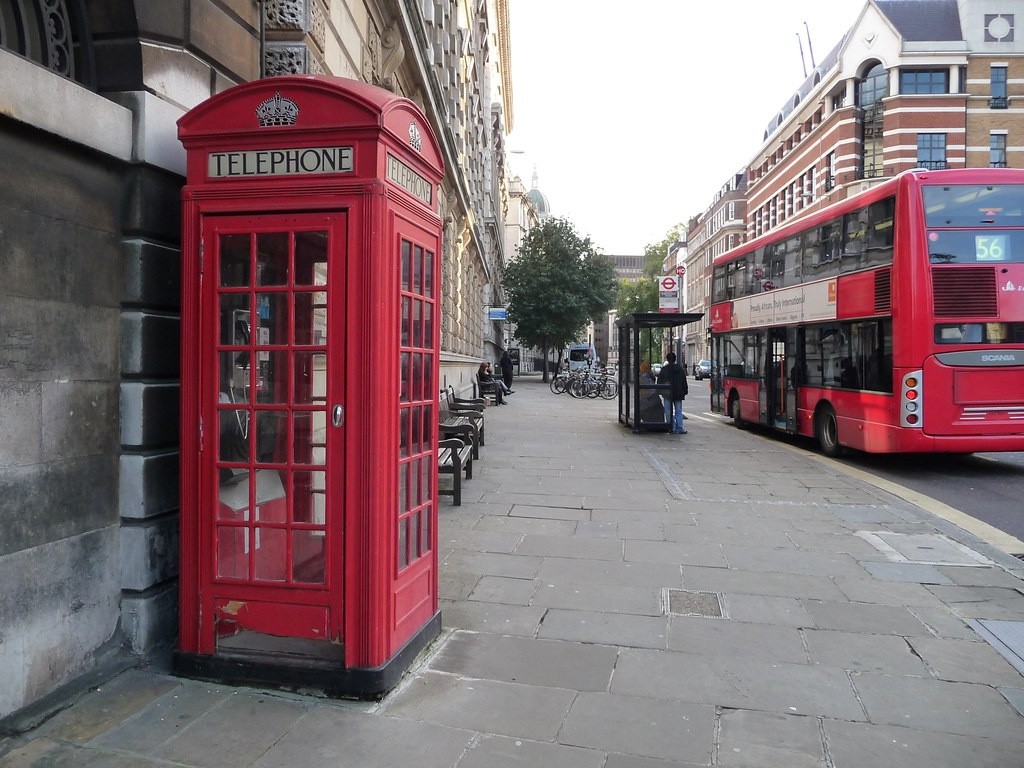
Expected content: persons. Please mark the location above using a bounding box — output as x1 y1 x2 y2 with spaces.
478 351 515 405
639 352 689 434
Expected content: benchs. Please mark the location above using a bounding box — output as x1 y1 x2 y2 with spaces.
439 391 485 460
439 423 479 506
476 374 499 406
444 385 486 441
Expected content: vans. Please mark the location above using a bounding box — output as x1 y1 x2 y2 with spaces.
569 344 596 371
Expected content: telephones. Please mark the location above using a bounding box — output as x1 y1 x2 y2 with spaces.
226 309 261 391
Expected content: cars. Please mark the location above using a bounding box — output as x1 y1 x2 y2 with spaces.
697 358 711 378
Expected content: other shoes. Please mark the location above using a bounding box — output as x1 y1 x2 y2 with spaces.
507 390 516 393
676 429 688 435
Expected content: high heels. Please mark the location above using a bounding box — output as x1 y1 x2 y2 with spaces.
504 391 512 397
499 399 508 406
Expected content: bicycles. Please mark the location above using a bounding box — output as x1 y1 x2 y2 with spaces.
550 369 617 400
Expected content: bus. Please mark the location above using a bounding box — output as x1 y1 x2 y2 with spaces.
711 168 1024 458
